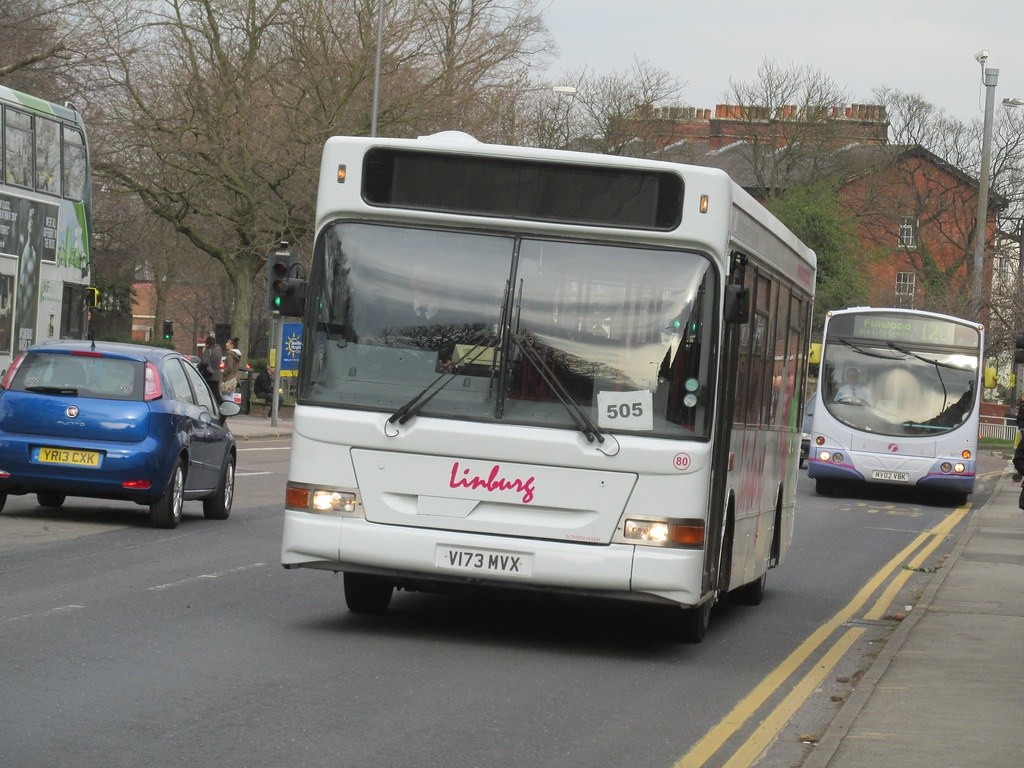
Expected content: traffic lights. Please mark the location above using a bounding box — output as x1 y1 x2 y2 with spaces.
268 248 295 312
163 320 172 341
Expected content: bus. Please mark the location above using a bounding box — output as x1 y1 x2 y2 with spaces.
1 86 101 384
805 306 1001 504
283 131 816 638
799 341 822 468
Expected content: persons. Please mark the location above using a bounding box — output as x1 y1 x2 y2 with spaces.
220 335 242 402
19 207 38 328
834 367 873 406
196 336 223 404
254 365 283 421
367 257 455 352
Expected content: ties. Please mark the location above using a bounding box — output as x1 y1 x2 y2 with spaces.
850 387 856 397
418 306 427 322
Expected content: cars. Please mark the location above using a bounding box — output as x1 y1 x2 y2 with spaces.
219 356 251 369
183 355 202 373
0 340 237 527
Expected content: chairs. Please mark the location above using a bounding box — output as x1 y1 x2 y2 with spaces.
49 359 86 389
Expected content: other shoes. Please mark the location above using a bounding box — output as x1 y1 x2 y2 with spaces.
268 416 282 420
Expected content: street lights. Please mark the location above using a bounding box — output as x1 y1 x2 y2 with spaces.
495 85 576 143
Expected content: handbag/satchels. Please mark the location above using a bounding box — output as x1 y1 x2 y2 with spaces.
220 377 237 394
198 349 214 378
